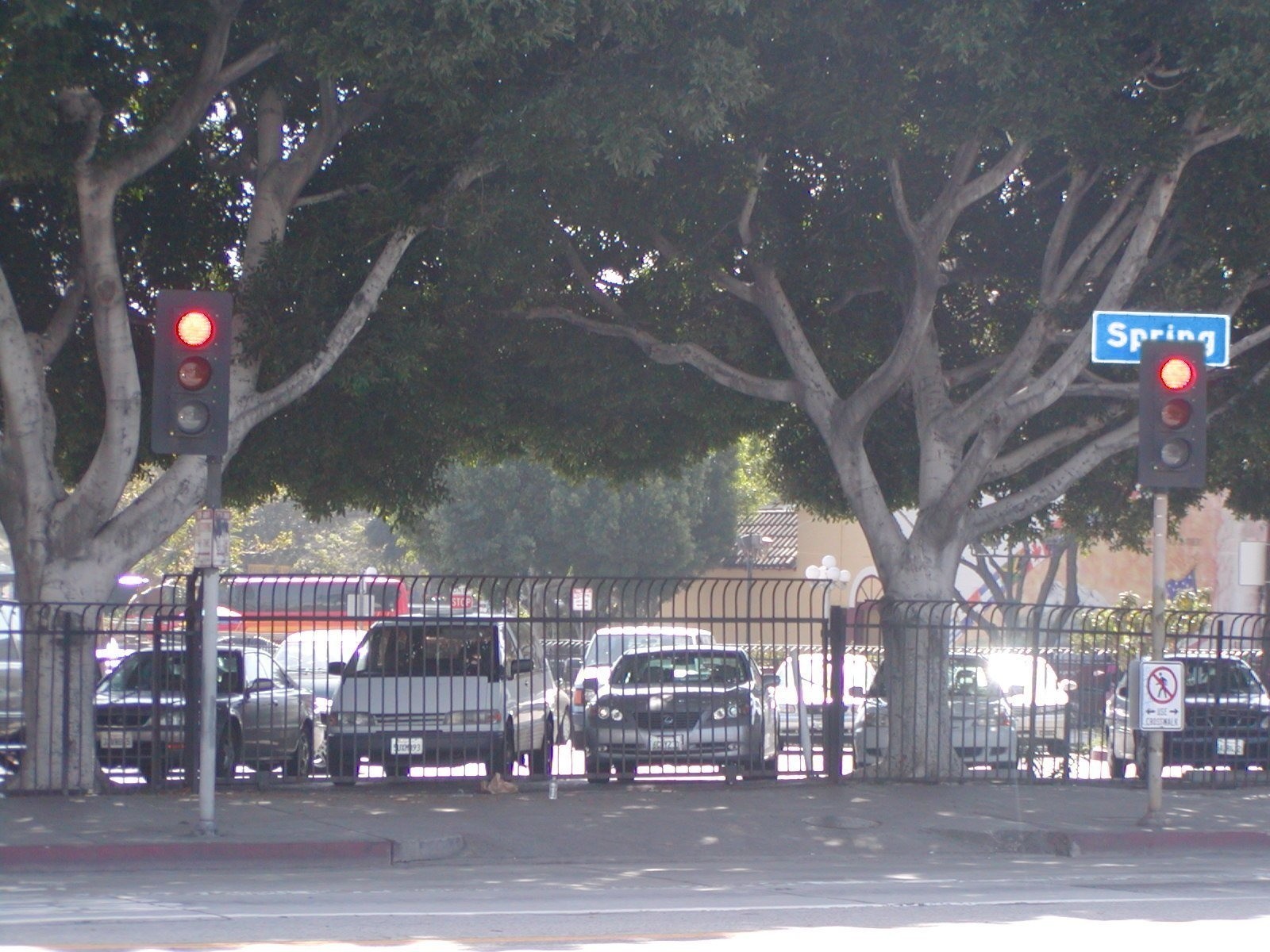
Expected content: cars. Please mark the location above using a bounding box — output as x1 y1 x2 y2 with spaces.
582 644 781 784
95 629 373 776
1105 653 1270 779
848 651 1025 770
775 654 875 750
554 677 572 746
982 653 1077 757
92 647 329 786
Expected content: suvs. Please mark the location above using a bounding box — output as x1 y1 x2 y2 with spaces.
0 600 33 772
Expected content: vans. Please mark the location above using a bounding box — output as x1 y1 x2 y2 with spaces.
326 610 558 786
563 626 717 753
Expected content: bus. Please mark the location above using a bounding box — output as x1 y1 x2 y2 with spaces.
111 575 408 655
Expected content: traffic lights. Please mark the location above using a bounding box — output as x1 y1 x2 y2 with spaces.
152 288 232 455
1139 339 1207 490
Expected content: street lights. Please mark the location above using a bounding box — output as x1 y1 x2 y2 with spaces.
736 530 760 596
805 555 850 646
